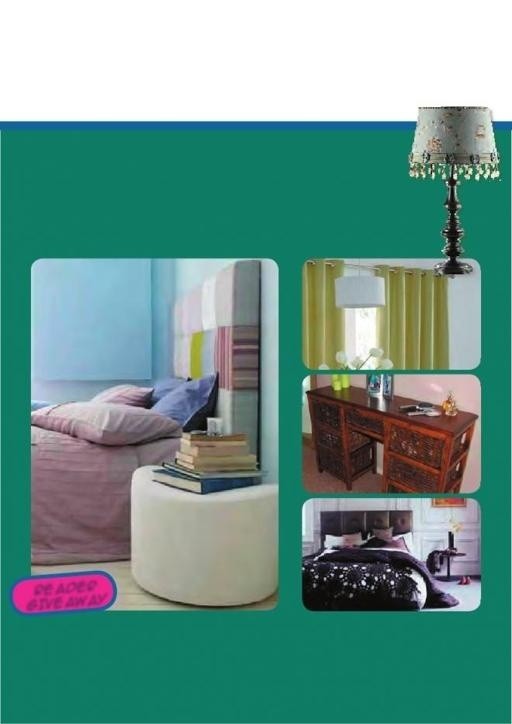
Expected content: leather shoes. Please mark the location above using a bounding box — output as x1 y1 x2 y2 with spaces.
458 576 470 585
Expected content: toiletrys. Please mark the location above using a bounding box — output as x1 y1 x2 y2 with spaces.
444 405 458 416
382 373 393 400
442 389 456 409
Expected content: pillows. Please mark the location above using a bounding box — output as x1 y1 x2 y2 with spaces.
30 371 221 447
323 525 415 556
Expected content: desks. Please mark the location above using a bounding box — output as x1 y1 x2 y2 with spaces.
435 553 467 582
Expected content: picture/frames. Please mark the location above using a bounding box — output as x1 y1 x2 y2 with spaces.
432 498 467 507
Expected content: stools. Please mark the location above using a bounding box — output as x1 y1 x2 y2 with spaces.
128 463 279 608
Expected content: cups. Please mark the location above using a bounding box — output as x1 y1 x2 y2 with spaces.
207 417 223 437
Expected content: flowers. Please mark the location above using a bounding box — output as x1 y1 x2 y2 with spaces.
318 347 394 370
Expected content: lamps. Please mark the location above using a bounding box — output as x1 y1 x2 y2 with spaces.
333 259 385 308
409 106 503 276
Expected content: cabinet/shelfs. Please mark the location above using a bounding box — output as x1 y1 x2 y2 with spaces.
305 385 478 494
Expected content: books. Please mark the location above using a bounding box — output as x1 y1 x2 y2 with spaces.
151 432 267 494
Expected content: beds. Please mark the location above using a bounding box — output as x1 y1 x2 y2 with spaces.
31 260 261 568
301 510 429 610
